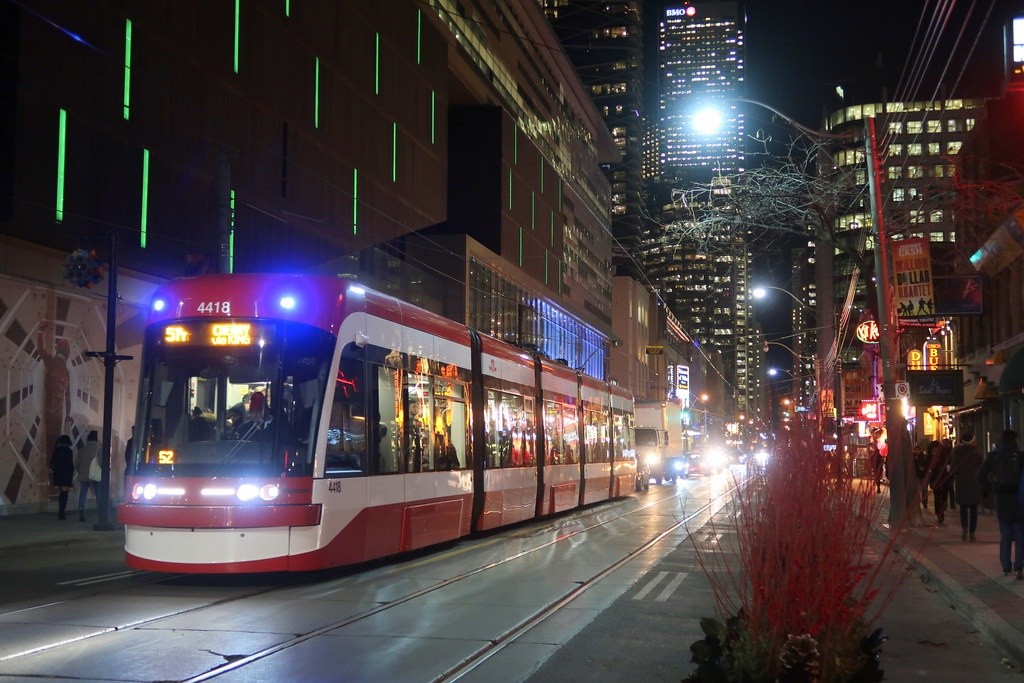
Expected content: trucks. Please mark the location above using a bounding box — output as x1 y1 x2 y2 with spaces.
634 400 683 484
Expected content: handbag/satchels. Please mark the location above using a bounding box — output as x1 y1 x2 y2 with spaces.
88 446 101 481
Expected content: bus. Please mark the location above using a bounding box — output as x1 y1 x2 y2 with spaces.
120 274 637 574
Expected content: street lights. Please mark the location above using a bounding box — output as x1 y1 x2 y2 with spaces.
751 286 843 487
690 98 907 530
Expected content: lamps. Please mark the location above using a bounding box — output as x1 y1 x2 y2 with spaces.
986 347 1008 364
974 378 991 398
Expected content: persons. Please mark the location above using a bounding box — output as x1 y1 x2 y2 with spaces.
189 385 292 444
585 440 610 462
913 439 956 525
408 400 460 470
976 430 1024 578
74 431 102 522
124 426 135 476
871 449 884 493
52 435 74 520
549 439 579 464
949 430 983 543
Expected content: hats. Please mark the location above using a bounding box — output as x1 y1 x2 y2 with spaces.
961 432 975 444
229 403 246 415
249 392 268 414
87 431 97 441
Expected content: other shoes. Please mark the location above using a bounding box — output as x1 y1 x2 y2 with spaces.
58 511 66 520
924 505 927 509
877 490 880 493
79 513 85 522
951 505 956 509
938 512 944 524
1005 568 1014 575
1015 570 1024 578
969 536 976 542
961 528 968 540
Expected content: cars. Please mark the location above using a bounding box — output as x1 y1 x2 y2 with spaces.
637 455 650 492
